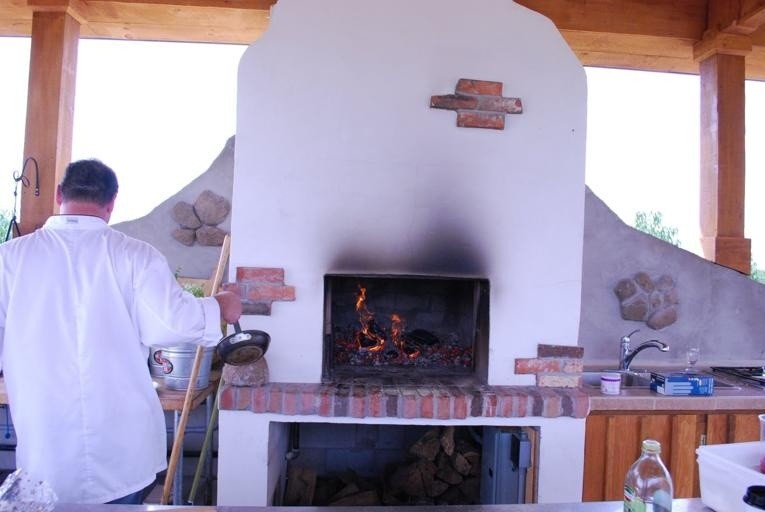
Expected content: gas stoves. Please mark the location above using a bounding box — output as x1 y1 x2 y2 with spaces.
709 366 765 387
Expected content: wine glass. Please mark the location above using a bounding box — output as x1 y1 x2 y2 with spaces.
684 345 701 374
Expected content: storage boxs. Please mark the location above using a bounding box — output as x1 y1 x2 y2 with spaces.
694 441 765 512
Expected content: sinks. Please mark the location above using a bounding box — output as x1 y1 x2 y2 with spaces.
582 368 653 389
633 370 742 391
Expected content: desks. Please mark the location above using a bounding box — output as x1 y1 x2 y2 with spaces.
0 497 725 512
0 373 222 505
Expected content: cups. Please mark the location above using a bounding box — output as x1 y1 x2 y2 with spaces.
600 376 621 395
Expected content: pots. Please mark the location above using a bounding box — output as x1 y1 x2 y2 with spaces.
216 321 271 367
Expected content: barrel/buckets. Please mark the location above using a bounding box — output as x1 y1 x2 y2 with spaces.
149 342 216 392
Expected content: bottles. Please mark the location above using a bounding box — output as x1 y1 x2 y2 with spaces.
622 439 675 511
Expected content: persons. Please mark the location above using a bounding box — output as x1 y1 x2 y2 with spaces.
0 158 243 506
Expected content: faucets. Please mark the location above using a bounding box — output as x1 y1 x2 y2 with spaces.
615 328 670 370
